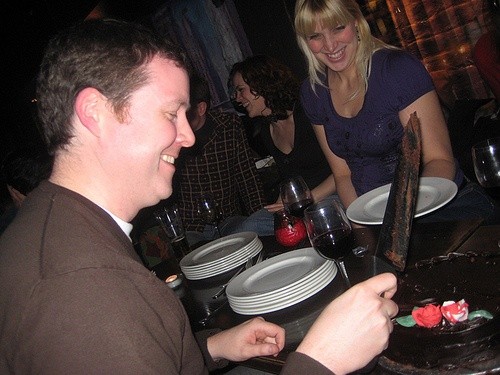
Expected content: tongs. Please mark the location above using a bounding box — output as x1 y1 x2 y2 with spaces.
212 253 263 299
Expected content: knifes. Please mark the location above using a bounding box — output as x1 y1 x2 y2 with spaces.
371 111 421 292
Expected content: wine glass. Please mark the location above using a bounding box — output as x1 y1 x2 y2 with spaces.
306 195 352 288
198 193 230 240
280 177 313 224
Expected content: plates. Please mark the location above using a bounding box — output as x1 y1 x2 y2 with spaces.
382 252 500 348
225 247 337 315
180 233 263 281
346 176 458 224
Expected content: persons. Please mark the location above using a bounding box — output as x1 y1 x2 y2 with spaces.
166 75 268 247
0 16 398 375
0 151 52 235
293 0 500 230
227 54 337 214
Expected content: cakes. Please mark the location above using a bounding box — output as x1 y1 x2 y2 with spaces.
383 251 500 372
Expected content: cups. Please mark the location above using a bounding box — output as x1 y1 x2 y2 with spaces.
154 198 190 261
473 139 500 187
274 209 306 248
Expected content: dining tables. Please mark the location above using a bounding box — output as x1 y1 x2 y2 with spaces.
149 216 500 375
